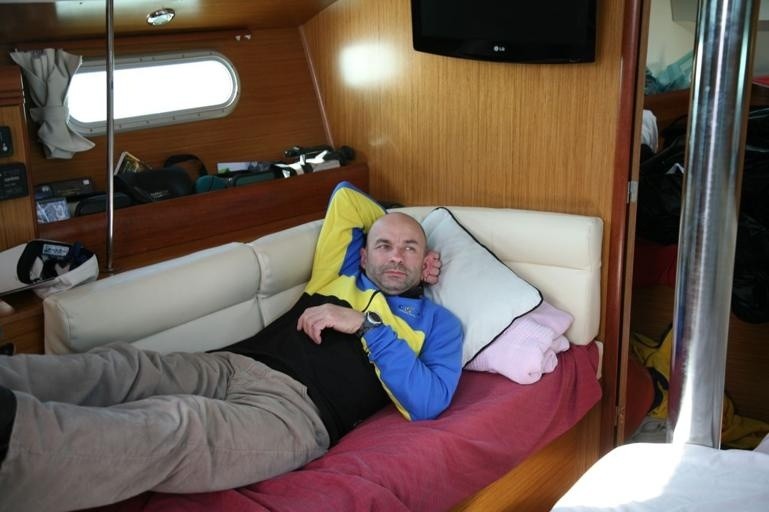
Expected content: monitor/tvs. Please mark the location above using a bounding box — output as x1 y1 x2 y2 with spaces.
410 0 597 64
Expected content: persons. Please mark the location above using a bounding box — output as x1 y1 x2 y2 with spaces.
1 179 466 511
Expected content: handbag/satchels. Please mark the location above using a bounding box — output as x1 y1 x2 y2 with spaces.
113 153 208 205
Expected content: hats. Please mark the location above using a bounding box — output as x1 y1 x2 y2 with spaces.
0 240 100 299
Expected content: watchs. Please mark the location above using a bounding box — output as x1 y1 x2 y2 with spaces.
353 311 382 340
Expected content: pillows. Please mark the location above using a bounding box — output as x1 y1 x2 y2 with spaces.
419 207 543 369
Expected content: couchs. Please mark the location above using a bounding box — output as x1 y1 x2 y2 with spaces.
42 205 605 512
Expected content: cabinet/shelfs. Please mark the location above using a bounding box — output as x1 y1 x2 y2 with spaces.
0 63 44 355
